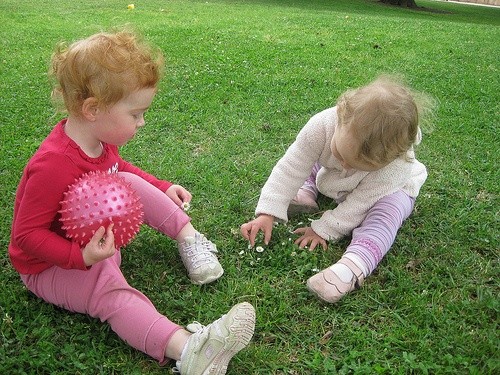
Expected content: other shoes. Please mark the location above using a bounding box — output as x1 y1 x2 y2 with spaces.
305 258 364 303
286 191 318 215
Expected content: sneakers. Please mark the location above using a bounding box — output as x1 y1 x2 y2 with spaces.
171 301 256 375
178 232 224 285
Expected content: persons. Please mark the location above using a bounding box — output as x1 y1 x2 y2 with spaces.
241 73 432 305
8 33 256 375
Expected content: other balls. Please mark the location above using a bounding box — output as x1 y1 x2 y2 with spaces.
58 169 146 250
